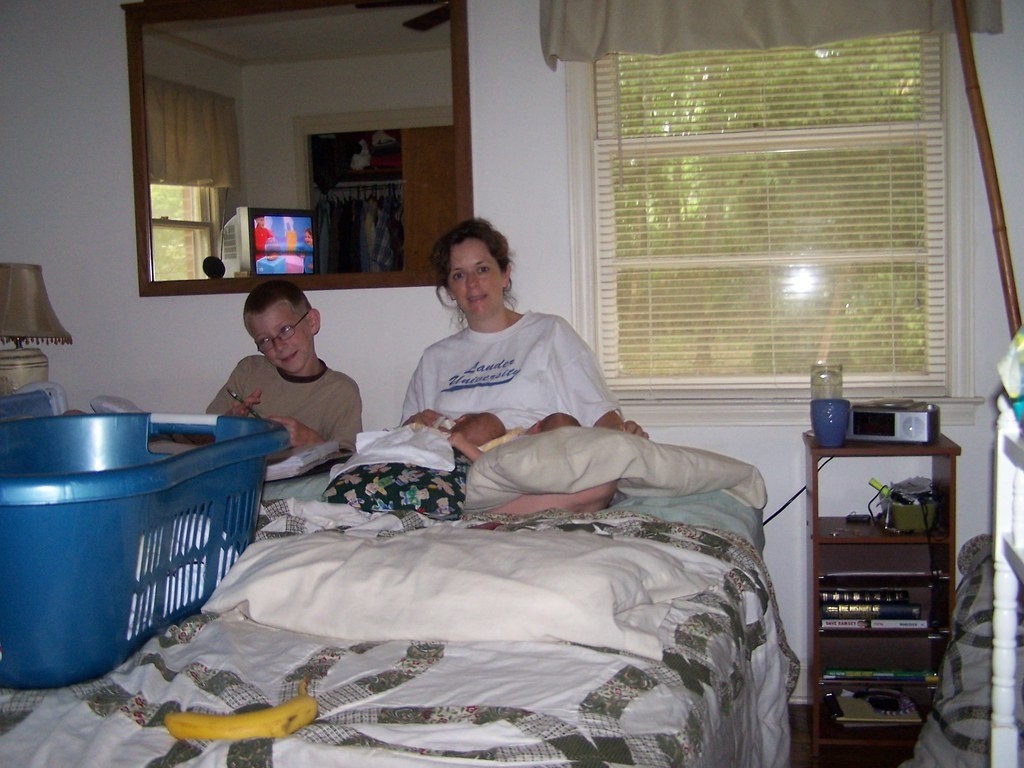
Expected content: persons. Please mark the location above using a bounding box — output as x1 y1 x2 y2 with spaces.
253 215 313 272
204 280 363 449
447 412 583 462
397 217 651 516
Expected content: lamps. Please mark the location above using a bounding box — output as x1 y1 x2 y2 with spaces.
0 263 72 398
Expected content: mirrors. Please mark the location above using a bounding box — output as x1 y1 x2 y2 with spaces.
120 0 473 298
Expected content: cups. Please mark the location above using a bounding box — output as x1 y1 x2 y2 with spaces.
813 399 851 448
810 363 843 400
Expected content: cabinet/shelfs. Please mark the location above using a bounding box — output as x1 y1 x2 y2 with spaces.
804 425 962 756
990 400 1024 768
295 108 455 275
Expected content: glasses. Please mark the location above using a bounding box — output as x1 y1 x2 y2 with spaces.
255 308 311 354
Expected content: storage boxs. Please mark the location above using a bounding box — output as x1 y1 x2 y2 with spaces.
0 414 290 690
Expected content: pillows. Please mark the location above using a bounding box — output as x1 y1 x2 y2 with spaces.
464 426 768 509
203 526 707 661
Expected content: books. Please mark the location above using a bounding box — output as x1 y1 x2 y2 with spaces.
264 438 355 481
819 588 939 728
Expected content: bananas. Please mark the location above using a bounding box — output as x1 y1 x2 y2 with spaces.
164 676 317 739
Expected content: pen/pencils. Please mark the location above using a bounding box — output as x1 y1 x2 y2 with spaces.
223 384 263 419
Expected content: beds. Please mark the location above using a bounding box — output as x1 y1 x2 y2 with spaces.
0 422 801 768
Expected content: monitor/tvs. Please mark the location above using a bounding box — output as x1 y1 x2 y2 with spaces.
217 207 319 277
